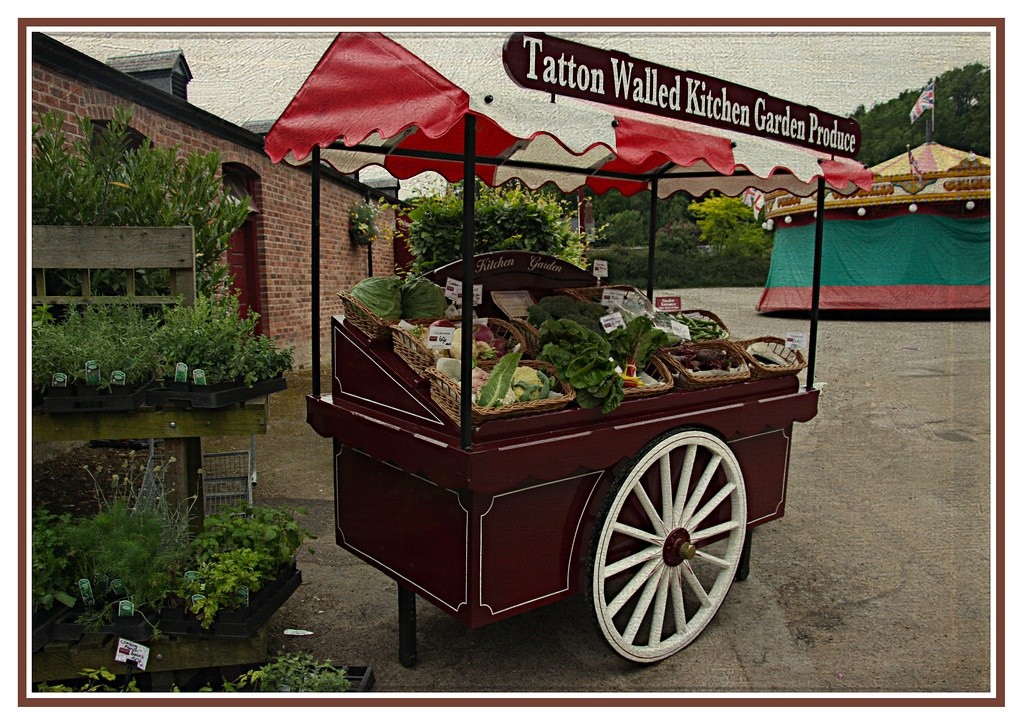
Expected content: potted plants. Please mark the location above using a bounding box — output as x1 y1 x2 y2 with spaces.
31 286 318 653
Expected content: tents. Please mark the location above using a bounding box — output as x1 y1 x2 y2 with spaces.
264 31 877 201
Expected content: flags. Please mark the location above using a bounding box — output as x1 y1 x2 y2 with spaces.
908 77 934 124
740 188 765 219
909 151 922 181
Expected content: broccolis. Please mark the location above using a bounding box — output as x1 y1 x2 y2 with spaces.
526 295 609 336
511 365 548 402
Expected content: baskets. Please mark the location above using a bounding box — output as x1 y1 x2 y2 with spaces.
336 284 809 432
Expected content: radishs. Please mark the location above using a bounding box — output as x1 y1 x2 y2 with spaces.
411 319 508 363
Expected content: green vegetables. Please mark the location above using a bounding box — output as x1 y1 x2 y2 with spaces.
541 316 666 414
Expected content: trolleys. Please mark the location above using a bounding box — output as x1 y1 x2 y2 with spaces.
263 32 887 673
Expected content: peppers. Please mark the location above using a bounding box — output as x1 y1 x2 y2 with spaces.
675 313 723 342
